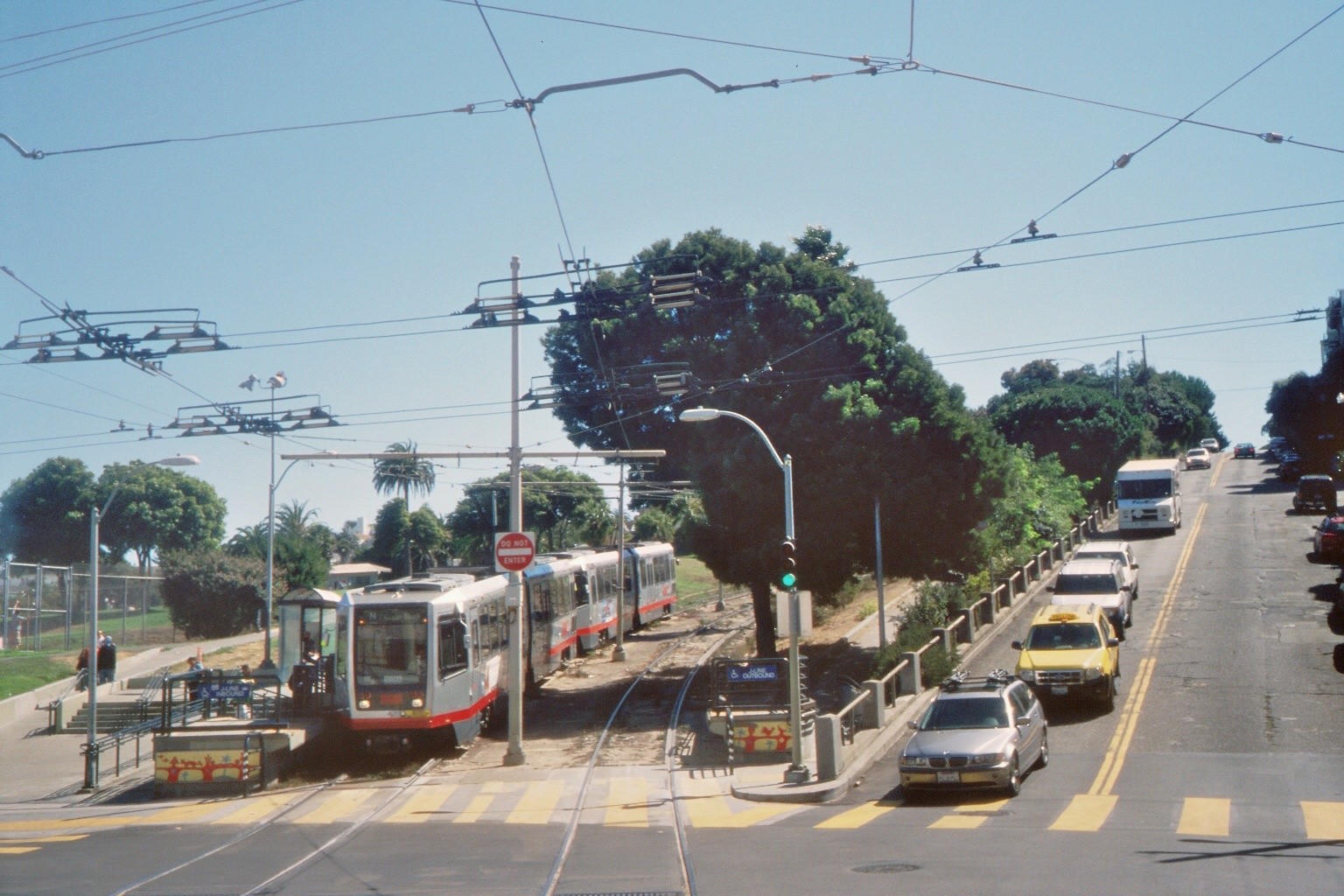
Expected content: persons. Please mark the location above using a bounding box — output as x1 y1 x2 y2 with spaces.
76 630 116 692
10 600 23 651
186 657 203 702
232 664 255 717
301 630 316 661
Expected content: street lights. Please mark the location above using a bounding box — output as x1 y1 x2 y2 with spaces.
254 376 341 679
677 404 809 784
78 453 200 794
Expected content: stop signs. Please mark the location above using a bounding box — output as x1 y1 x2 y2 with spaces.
493 532 535 574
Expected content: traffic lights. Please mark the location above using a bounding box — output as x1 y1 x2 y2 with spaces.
779 538 797 589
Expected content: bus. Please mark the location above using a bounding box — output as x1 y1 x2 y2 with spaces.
1114 459 1181 537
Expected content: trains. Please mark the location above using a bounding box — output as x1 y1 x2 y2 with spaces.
336 486 677 764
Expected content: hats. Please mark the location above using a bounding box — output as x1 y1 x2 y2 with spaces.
105 636 112 647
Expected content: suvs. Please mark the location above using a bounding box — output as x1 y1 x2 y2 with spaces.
1292 475 1337 513
1068 541 1140 601
1046 558 1133 641
1013 602 1119 715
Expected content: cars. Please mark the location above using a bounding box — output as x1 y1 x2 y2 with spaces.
1334 569 1344 611
1269 435 1301 478
1200 439 1220 453
1233 442 1256 459
1184 448 1212 469
898 670 1049 801
1311 515 1344 561
